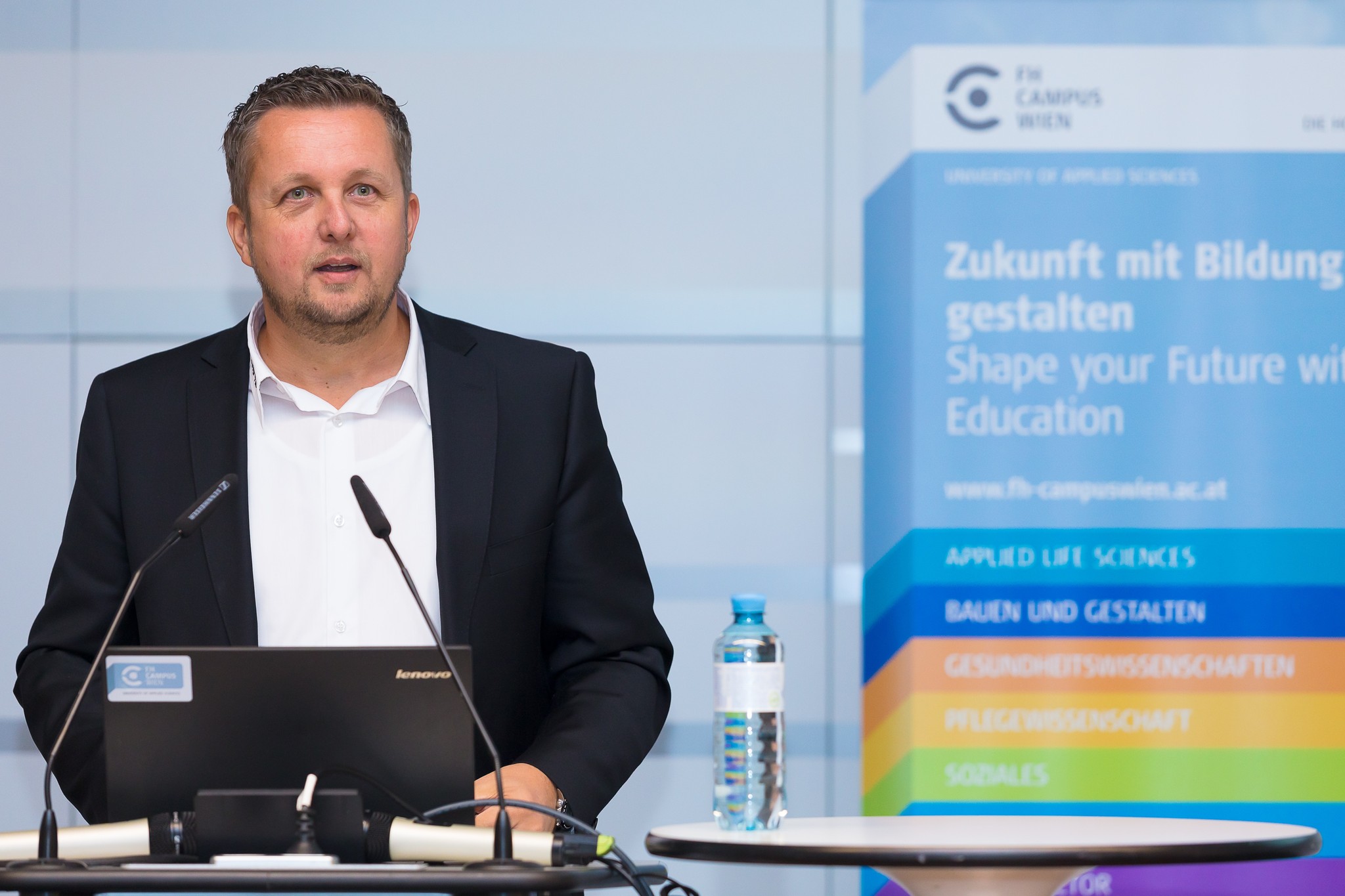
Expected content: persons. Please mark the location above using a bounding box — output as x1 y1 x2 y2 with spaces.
15 67 673 869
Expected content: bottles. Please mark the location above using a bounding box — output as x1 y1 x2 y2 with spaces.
713 593 785 834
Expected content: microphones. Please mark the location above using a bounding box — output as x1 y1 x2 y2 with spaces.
10 468 237 871
350 475 535 871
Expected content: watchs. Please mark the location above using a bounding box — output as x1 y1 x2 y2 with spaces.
556 787 575 833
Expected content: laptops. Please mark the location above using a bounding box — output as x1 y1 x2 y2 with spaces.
99 645 476 865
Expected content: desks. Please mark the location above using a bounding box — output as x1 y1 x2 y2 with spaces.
648 813 1322 896
